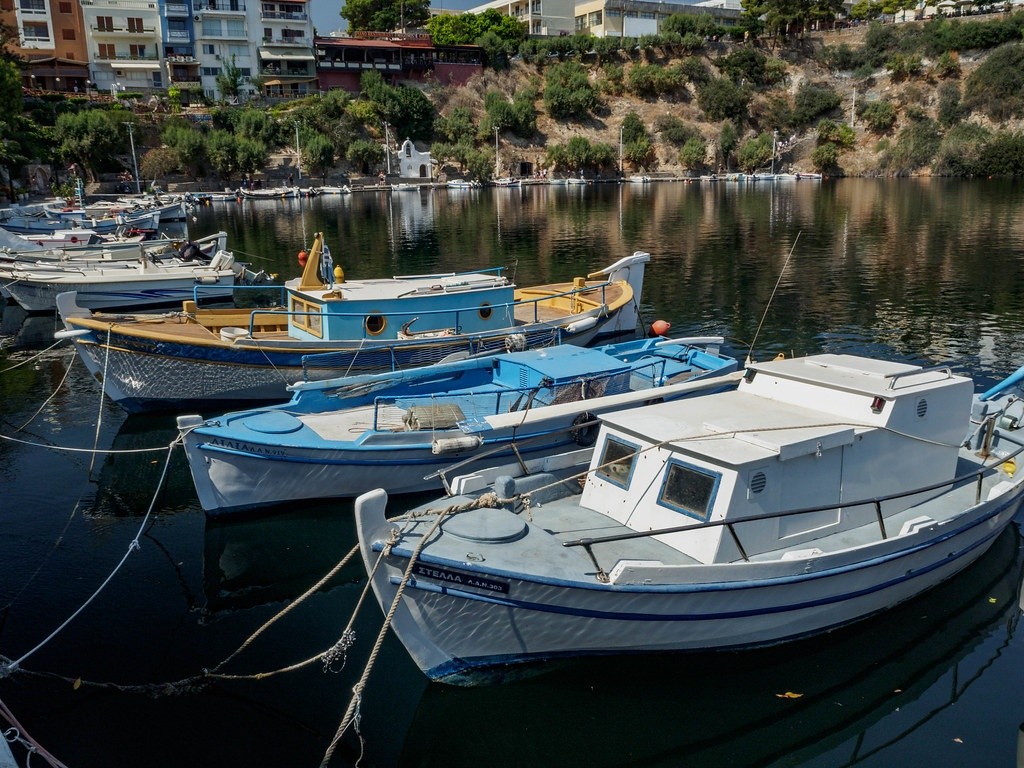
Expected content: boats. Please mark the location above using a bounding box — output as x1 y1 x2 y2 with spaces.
239 187 299 199
550 180 566 184
354 353 1024 684
569 179 587 184
495 177 521 187
705 173 776 181
0 230 267 310
391 183 418 191
197 488 446 621
0 193 238 231
176 319 737 516
6 296 238 361
321 185 352 194
447 180 477 187
395 520 1024 768
54 250 651 413
80 413 219 522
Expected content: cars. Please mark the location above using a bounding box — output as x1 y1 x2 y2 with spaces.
117 172 132 181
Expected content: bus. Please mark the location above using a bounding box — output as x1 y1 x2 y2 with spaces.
351 184 364 190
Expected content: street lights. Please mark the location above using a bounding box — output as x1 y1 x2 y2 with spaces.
382 121 391 173
495 123 500 178
619 122 626 172
294 121 302 179
128 124 141 194
2 138 16 204
